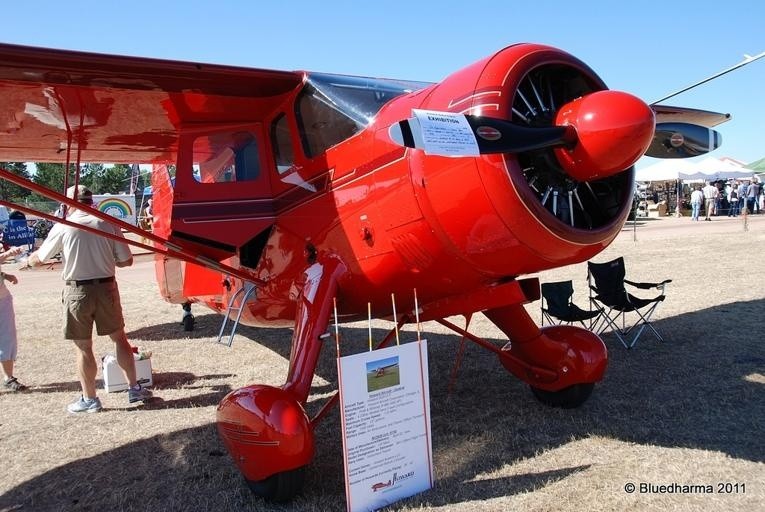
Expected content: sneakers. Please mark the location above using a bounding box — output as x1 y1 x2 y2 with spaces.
129 384 153 403
67 394 103 413
4 376 26 390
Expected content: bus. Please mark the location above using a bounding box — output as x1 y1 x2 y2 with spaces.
681 175 764 211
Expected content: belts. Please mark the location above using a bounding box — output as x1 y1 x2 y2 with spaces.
66 277 114 286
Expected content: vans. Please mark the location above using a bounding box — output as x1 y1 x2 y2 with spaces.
0 205 7 233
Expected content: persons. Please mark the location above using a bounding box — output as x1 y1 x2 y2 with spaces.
28 184 155 412
0 206 26 391
688 181 765 222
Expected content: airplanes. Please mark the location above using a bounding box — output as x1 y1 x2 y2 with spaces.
369 363 399 380
0 37 765 508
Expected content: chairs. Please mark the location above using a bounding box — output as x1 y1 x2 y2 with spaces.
540 278 605 331
585 256 673 351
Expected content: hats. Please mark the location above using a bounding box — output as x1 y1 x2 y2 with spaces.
67 185 93 199
